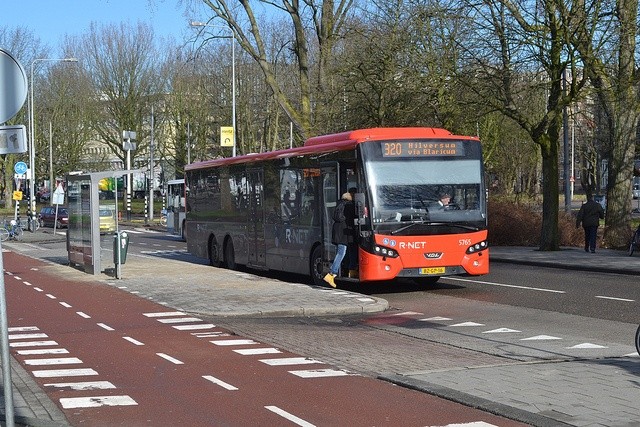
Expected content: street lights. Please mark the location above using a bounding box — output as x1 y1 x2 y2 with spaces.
30 58 78 231
191 21 238 159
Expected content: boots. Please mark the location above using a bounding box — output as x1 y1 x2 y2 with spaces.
348 270 358 277
323 273 337 287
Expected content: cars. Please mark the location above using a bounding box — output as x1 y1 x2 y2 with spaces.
100 207 115 235
38 208 69 228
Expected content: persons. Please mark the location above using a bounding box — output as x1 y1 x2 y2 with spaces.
432 192 452 209
576 193 604 252
322 187 359 289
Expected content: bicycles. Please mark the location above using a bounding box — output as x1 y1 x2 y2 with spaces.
0 218 23 241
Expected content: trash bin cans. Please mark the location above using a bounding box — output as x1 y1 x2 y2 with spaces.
112 230 129 264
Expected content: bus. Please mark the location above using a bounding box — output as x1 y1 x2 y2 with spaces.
184 127 489 289
166 178 185 243
